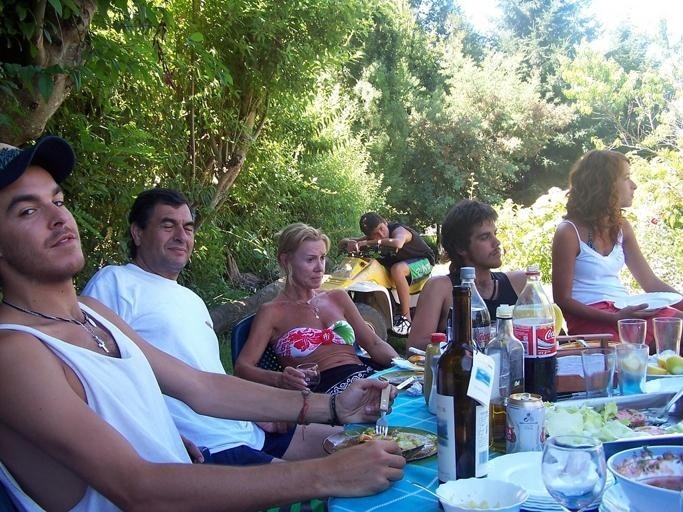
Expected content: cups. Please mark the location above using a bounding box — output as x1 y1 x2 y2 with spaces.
615 343 650 395
617 319 648 347
581 348 616 398
653 315 682 363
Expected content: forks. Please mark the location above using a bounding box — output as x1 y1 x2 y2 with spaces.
644 388 683 425
375 382 392 437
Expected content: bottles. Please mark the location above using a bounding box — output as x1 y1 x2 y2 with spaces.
423 264 557 485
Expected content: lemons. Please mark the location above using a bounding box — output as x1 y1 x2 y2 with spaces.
618 353 683 378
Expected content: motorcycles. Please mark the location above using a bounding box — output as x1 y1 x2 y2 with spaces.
319 245 436 343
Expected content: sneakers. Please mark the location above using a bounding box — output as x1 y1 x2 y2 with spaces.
392 313 412 336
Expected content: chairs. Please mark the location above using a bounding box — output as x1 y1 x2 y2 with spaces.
231 310 391 387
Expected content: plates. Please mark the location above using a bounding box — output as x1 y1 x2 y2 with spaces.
613 291 683 312
322 425 438 461
487 450 630 512
378 369 426 386
646 375 683 381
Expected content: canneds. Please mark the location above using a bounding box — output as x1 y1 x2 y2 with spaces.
504 392 545 451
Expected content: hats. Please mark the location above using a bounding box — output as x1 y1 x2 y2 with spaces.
2 137 78 190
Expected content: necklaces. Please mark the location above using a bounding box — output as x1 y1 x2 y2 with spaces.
4 298 111 355
477 272 497 301
287 291 320 320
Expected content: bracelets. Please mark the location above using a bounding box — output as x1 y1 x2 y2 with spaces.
296 388 312 426
328 394 341 425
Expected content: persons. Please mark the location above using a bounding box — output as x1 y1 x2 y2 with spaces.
80 188 345 465
0 135 407 512
406 200 568 353
551 149 683 354
233 222 400 396
337 211 438 338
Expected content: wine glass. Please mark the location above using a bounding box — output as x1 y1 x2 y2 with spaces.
296 362 321 393
541 434 607 512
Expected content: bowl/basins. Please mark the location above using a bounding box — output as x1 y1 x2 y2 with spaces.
436 477 531 512
606 445 683 512
